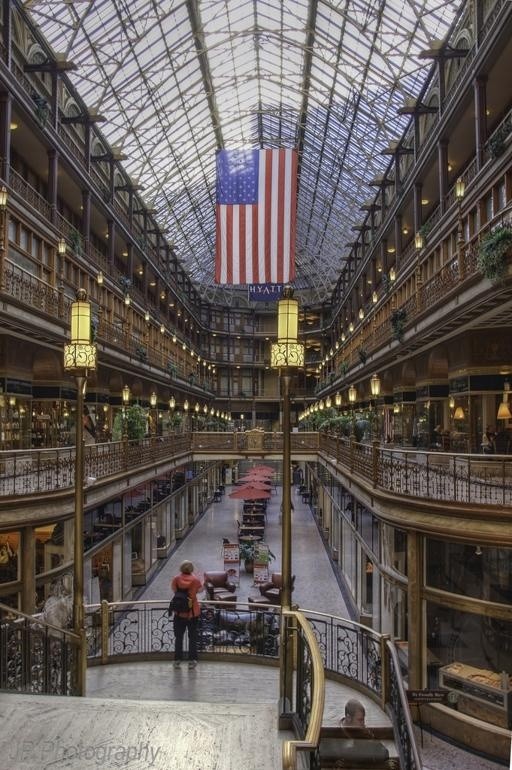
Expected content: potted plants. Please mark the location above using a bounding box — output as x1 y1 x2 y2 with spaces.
240 544 275 573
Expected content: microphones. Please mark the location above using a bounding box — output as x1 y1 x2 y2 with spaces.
248 596 270 611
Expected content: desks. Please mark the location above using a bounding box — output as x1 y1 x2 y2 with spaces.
315 265 397 444
454 174 468 282
240 501 266 544
122 295 212 440
413 233 424 312
270 285 305 712
63 289 97 698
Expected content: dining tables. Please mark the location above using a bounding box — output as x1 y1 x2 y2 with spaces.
240 544 275 573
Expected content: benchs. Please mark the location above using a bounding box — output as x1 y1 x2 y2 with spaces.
214 146 298 286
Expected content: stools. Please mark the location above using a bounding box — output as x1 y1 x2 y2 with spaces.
248 596 270 611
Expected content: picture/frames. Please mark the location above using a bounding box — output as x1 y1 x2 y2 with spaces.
240 501 266 544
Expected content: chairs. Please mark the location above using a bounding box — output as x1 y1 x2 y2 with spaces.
203 571 237 609
237 520 243 536
260 572 296 606
173 658 198 669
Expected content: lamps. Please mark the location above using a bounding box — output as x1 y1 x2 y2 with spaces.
297 179 465 419
237 520 243 536
0 186 231 422
497 402 511 420
260 572 296 606
203 571 237 609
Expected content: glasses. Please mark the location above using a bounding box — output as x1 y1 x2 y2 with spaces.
497 402 511 420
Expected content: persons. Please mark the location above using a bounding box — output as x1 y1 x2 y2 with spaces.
338 699 365 726
169 560 204 669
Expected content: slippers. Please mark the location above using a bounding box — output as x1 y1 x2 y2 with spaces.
169 580 194 613
